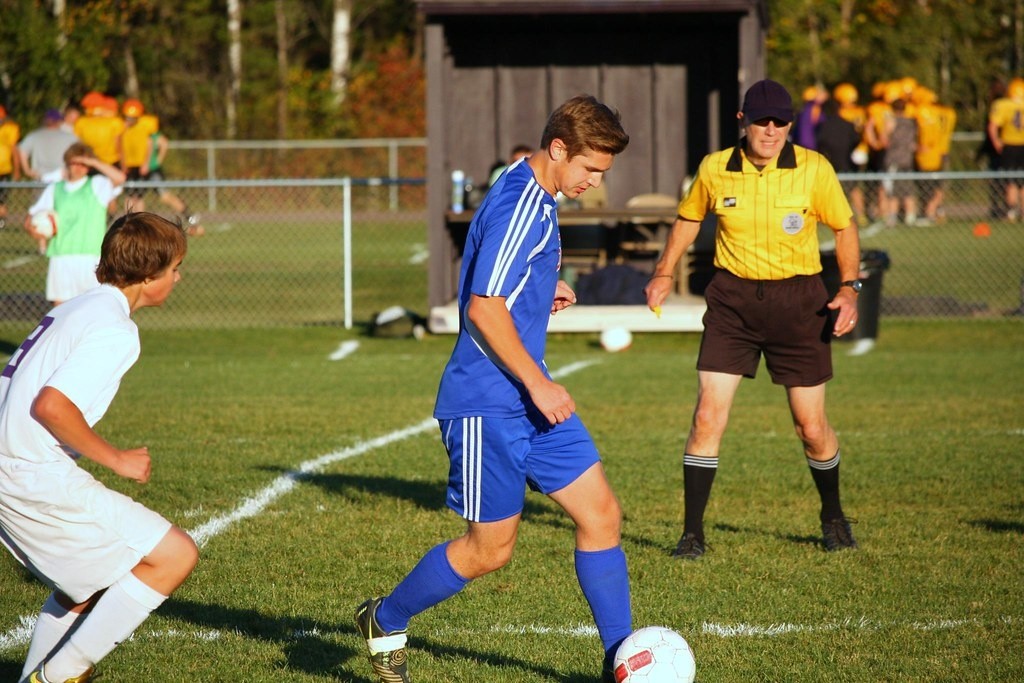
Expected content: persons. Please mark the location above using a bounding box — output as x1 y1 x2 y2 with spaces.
467 145 611 296
644 79 863 560
353 95 633 683
0 211 199 683
988 76 1024 222
25 144 127 308
0 106 21 229
17 91 203 236
790 75 960 226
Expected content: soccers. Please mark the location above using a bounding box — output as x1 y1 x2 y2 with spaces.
611 624 698 683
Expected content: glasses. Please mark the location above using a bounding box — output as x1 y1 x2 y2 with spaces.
753 117 789 128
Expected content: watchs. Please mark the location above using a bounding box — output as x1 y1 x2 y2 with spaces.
838 279 863 293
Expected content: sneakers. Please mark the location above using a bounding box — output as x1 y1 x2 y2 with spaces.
818 512 859 553
354 596 408 683
21 661 103 683
671 533 714 558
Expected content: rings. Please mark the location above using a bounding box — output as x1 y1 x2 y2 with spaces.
850 321 856 327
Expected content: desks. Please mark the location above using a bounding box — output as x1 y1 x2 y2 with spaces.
448 208 697 301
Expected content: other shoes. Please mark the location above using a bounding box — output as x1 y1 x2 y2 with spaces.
1002 213 1024 223
855 212 947 227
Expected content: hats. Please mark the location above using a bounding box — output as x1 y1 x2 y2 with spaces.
740 79 793 129
873 77 938 104
82 92 144 118
834 84 857 103
1008 79 1024 98
44 109 64 124
803 88 820 101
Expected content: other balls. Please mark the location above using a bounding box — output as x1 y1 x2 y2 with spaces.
31 210 58 240
